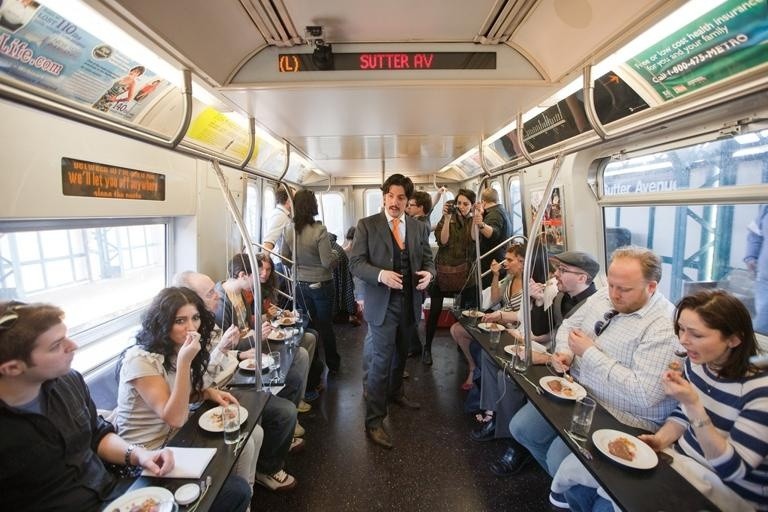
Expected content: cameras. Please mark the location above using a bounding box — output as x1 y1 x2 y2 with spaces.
447 205 457 214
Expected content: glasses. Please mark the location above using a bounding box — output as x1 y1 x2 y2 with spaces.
594 309 620 337
555 263 590 280
407 203 418 208
0 300 30 334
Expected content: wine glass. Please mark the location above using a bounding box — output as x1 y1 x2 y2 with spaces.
267 351 281 381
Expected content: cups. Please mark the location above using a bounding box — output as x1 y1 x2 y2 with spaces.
294 309 304 324
514 338 532 377
221 405 241 445
452 293 463 313
285 327 294 347
489 328 500 352
572 397 596 440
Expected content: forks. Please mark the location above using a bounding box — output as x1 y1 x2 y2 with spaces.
564 426 593 462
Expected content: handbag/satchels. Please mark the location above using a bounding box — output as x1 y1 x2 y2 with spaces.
436 262 472 293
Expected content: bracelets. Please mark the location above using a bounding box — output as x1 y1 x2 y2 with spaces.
689 417 711 429
124 444 140 467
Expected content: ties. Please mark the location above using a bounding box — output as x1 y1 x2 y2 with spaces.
392 217 403 250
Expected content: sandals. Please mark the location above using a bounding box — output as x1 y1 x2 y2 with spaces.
472 409 495 423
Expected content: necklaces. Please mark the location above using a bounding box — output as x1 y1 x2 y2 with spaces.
705 366 721 394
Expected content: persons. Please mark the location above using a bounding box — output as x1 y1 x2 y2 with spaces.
510 245 681 478
213 172 548 447
487 250 601 478
744 204 768 337
172 271 306 492
0 0 34 31
133 79 160 103
111 285 265 511
91 66 145 113
0 300 251 512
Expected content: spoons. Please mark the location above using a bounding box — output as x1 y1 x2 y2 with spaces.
555 351 574 384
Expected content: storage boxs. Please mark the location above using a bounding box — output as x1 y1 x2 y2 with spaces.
423 297 456 327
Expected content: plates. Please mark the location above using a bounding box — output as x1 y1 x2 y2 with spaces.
101 486 173 511
503 343 538 359
590 428 660 470
198 404 249 432
267 315 299 341
538 375 586 403
462 309 507 333
237 358 270 370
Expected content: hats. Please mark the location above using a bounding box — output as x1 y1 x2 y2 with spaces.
553 250 600 279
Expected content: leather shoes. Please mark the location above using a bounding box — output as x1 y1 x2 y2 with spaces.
460 382 474 390
489 442 533 477
363 342 422 399
366 425 393 449
393 394 421 409
469 416 496 442
348 315 362 326
423 345 432 365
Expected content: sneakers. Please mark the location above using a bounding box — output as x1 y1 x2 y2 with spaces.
549 491 570 509
294 420 305 436
296 369 338 414
288 437 305 454
255 467 296 491
